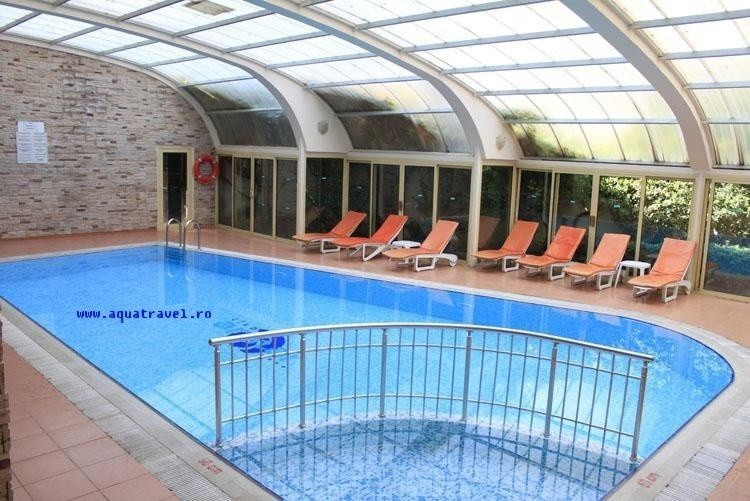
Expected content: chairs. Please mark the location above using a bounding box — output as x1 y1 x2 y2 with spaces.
627 237 697 303
515 225 586 281
470 220 539 273
562 232 631 290
292 209 367 254
320 214 408 261
381 219 458 272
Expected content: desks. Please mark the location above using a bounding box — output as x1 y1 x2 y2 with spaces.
614 260 652 289
392 240 421 247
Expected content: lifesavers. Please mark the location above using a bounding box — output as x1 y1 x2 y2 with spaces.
193 155 219 185
